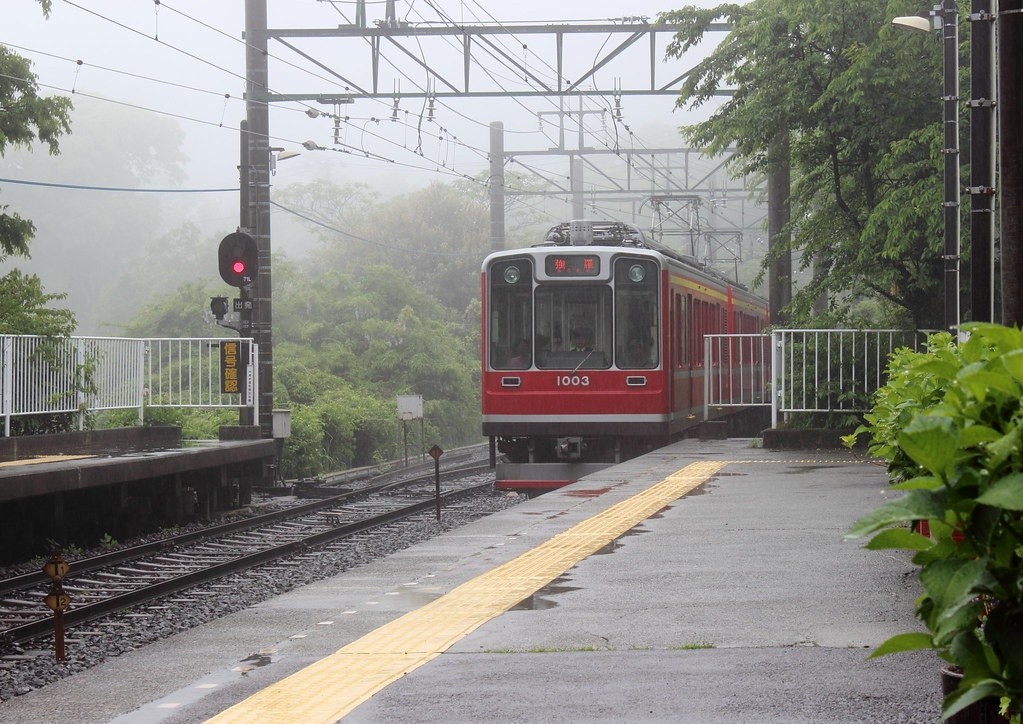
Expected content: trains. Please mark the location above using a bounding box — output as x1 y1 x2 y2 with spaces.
481 220 772 499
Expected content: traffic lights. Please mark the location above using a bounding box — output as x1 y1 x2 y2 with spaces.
218 232 258 287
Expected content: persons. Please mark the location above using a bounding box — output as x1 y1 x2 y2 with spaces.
628 339 647 360
508 340 529 369
571 335 593 351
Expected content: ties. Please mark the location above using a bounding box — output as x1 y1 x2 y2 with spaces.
578 348 582 352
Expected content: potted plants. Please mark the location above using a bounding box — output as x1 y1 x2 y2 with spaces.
840 324 1023 724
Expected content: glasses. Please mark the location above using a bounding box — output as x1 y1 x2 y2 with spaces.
573 335 586 339
